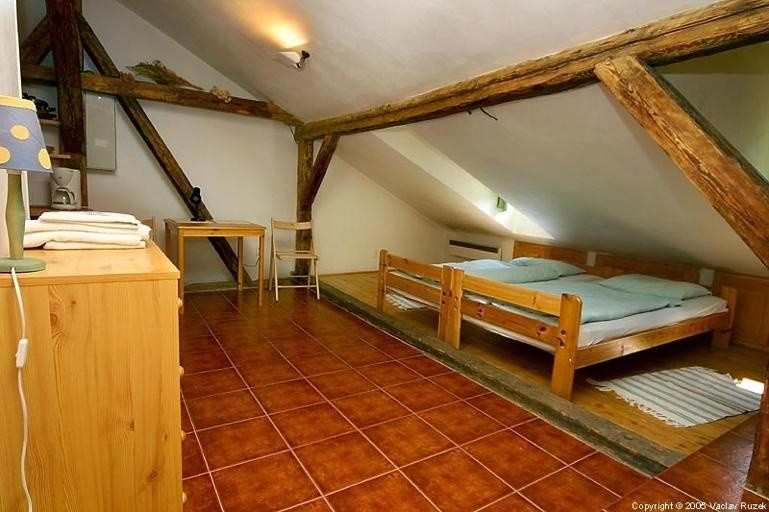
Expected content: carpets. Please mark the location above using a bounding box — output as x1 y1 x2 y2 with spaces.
586 366 763 429
385 291 426 310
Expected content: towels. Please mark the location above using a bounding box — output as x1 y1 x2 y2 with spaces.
23 210 150 251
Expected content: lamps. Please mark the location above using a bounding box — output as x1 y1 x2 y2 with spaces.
0 94 55 272
278 51 303 71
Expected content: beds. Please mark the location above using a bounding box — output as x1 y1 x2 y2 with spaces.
444 257 739 402
376 249 606 341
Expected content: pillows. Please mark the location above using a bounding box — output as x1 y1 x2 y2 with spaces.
598 274 713 301
510 257 587 277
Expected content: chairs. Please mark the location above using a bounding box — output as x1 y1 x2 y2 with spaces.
143 216 156 244
268 218 319 302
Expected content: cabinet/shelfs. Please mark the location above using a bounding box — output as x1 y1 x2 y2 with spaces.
26 118 93 217
1 239 188 512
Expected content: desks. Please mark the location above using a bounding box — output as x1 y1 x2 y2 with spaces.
163 218 267 315
